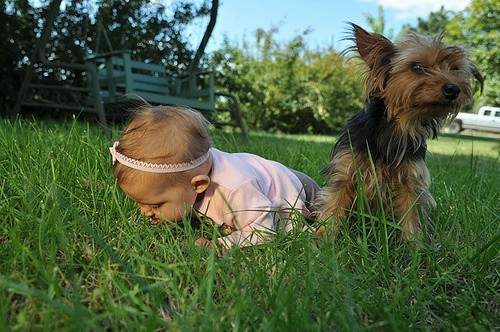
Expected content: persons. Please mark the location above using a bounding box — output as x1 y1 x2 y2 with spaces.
109 93 323 257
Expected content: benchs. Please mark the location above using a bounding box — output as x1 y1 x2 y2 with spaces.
82 52 215 111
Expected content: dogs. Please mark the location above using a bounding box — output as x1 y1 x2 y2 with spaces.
306 20 484 245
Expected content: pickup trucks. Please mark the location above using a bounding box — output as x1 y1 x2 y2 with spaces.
447 106 500 134
